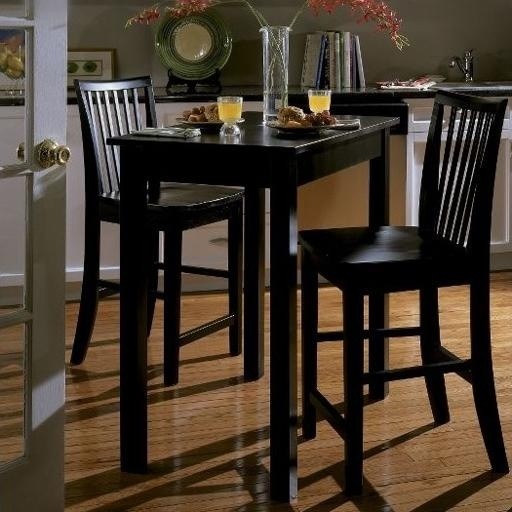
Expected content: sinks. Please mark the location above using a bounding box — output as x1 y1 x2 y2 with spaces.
432 81 512 92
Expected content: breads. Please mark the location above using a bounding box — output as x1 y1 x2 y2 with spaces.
205 103 218 123
278 105 304 125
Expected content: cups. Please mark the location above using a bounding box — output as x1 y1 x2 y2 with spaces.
307 89 334 114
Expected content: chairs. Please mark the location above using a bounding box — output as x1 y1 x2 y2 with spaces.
298 89 509 496
73 73 243 386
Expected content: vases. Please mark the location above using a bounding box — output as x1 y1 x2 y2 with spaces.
259 26 293 122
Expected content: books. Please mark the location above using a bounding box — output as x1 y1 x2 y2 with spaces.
299 30 366 91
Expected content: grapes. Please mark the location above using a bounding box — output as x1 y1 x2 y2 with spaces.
301 110 336 126
182 105 204 122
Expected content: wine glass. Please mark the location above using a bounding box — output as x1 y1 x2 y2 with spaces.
216 96 243 137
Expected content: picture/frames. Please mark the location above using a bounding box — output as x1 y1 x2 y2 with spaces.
67 48 118 89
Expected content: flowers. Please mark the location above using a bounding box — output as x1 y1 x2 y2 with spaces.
124 1 409 107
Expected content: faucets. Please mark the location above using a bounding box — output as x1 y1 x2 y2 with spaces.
447 47 475 83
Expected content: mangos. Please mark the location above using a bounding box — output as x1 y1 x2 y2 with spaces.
0 49 24 77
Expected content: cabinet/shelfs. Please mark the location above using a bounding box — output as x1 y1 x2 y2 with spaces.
390 88 512 272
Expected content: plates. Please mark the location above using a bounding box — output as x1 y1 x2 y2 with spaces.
154 9 233 81
176 119 245 130
428 75 446 83
265 122 336 137
